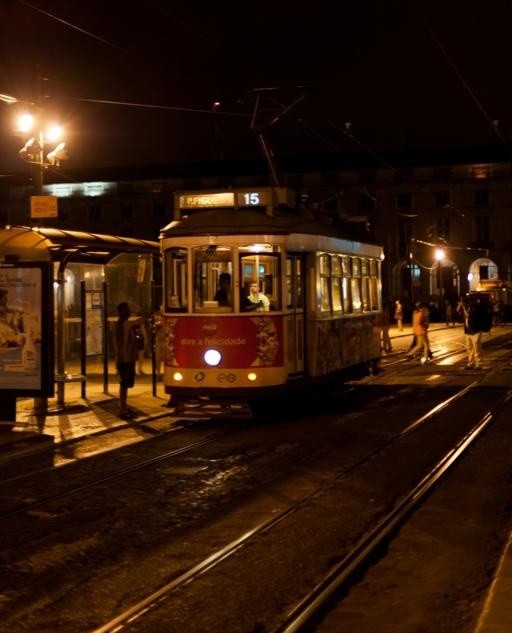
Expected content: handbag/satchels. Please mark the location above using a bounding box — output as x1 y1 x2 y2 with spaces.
132 332 145 353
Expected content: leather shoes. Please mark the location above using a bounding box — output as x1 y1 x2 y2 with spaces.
464 366 481 370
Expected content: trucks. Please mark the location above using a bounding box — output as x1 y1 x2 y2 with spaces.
466 278 512 326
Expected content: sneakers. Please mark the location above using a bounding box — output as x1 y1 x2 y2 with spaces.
406 355 433 365
381 347 393 352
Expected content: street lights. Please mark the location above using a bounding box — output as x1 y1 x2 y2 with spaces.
11 107 71 224
435 246 445 323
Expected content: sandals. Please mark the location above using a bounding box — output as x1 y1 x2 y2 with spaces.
118 409 136 419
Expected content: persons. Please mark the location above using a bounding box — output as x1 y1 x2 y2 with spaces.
113 301 146 411
152 301 164 376
381 302 393 352
444 299 455 326
394 299 404 331
404 299 431 367
456 292 482 370
213 271 270 312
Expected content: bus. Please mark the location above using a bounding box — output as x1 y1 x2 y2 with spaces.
151 185 390 421
